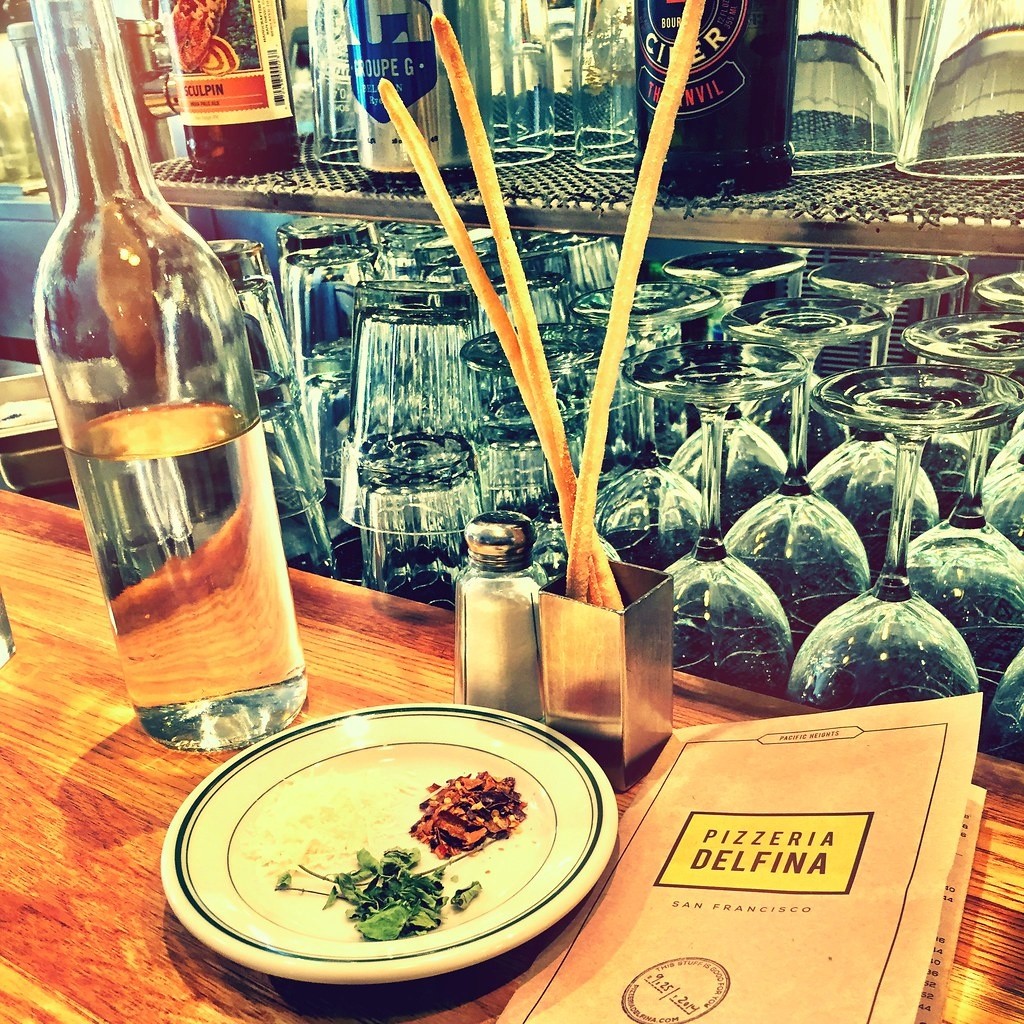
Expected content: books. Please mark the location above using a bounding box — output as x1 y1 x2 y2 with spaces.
495 692 986 1024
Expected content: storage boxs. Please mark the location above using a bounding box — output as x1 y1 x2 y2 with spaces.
538 563 677 798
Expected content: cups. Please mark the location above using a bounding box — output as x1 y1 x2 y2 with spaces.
308 0 640 169
788 0 905 176
894 0 1024 180
182 209 636 617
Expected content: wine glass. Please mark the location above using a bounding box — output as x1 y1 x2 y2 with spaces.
630 250 1023 768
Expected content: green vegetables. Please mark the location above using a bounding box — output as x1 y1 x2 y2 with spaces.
275 847 482 941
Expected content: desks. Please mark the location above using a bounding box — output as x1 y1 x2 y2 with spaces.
0 450 1024 1024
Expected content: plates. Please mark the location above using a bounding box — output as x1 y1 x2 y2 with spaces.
159 702 618 983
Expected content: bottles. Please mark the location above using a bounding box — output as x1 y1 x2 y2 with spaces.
631 0 794 198
161 0 304 176
21 0 312 755
453 512 554 721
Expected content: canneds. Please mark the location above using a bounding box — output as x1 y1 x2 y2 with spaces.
344 0 451 173
